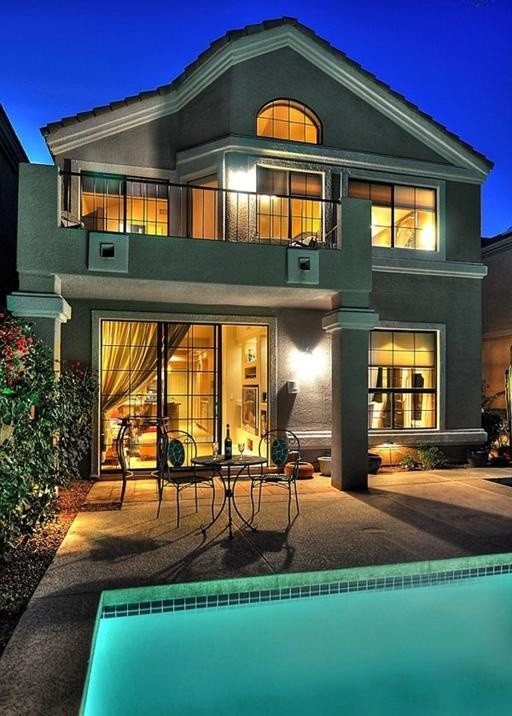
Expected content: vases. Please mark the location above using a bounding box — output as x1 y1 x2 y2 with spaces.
284 462 314 478
368 453 382 474
317 456 332 476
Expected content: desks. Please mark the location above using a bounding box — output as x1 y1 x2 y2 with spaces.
190 455 269 538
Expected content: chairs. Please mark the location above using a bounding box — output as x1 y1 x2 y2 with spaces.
247 428 302 525
156 430 215 529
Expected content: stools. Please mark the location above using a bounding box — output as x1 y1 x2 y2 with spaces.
138 432 158 460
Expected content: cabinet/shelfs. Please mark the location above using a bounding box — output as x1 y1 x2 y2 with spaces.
115 414 171 518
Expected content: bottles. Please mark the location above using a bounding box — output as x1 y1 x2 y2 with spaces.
224 424 232 460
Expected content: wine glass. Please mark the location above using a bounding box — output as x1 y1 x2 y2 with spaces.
212 442 219 461
238 442 245 461
